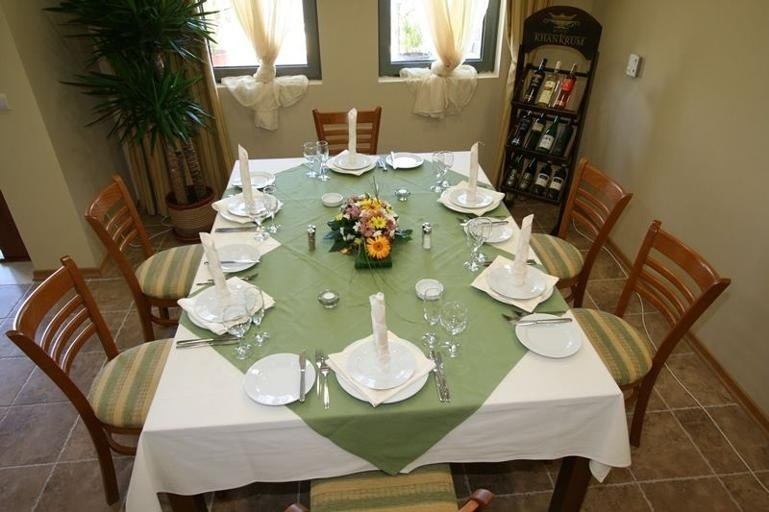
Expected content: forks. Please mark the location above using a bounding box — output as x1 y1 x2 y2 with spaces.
320 353 330 410
501 314 519 321
315 349 323 400
456 217 509 226
517 318 573 326
510 309 565 316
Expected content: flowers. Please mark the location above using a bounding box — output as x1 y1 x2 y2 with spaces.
334 191 400 256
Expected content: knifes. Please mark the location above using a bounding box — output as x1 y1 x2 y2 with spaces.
299 350 306 404
204 259 260 264
391 150 397 170
177 335 240 344
176 340 240 349
430 351 444 402
436 351 452 402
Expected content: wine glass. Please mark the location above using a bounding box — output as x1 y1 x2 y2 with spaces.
421 288 440 348
304 142 316 180
439 302 468 357
248 200 270 240
222 304 255 360
243 286 273 347
473 218 491 263
463 222 480 272
262 185 281 234
440 152 455 188
430 154 444 192
316 141 330 182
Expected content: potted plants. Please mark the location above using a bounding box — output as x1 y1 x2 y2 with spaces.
39 0 214 241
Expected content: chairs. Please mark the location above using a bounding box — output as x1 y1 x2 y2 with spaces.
285 463 493 512
85 175 206 341
570 219 732 448
531 158 632 306
6 255 173 506
312 106 382 155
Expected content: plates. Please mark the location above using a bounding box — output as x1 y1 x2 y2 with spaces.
244 353 316 405
464 217 513 243
488 265 546 298
219 199 280 223
386 152 424 169
238 170 275 189
228 194 254 216
217 243 260 273
336 371 430 404
539 286 554 304
516 313 581 358
340 341 416 390
194 284 255 322
187 312 210 329
333 154 371 169
449 189 494 208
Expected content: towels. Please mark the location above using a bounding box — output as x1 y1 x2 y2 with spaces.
237 144 254 204
513 212 536 284
470 253 560 314
211 188 284 225
369 293 390 363
326 149 381 176
435 179 508 217
466 142 480 196
197 231 231 298
325 328 438 409
177 274 277 336
348 109 355 155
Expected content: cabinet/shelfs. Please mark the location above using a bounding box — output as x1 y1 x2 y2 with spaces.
500 5 602 235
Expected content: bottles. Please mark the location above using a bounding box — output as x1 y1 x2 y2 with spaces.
306 224 316 250
535 114 560 153
522 58 547 103
317 289 338 309
504 154 524 187
547 164 567 199
522 111 547 149
549 117 574 155
551 63 579 108
531 160 553 194
518 157 538 190
534 60 562 107
421 223 433 250
394 188 411 202
510 110 533 146
